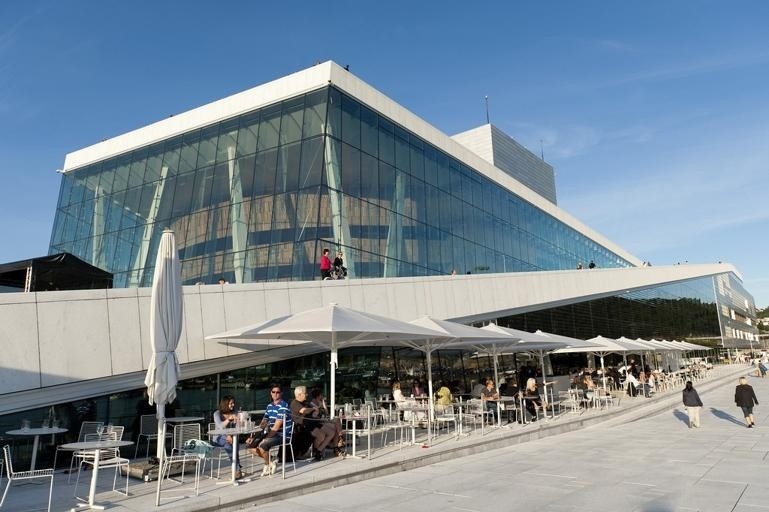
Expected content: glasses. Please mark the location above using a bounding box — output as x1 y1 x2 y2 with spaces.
272 391 281 394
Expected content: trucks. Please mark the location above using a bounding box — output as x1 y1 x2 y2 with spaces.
681 356 713 370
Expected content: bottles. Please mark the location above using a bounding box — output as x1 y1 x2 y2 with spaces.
377 393 390 403
397 393 428 410
338 401 369 417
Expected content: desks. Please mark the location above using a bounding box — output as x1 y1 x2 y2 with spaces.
58 440 135 512
5 428 69 487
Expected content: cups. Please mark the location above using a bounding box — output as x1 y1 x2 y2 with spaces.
41 418 61 431
21 418 32 434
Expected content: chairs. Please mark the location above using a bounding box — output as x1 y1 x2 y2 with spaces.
54 421 125 484
74 432 130 503
2 444 54 512
289 384 543 459
134 404 298 497
541 363 711 423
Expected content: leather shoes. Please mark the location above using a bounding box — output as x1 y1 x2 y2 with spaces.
235 471 242 480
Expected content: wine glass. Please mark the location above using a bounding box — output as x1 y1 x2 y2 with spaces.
94 422 114 444
228 412 257 433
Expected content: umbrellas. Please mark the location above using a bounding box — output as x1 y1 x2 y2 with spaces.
471 322 566 425
401 316 522 421
524 329 607 405
144 227 183 460
553 336 714 395
204 303 454 421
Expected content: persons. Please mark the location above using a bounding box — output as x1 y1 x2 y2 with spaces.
735 377 759 428
392 360 540 424
218 279 229 284
309 389 346 457
577 263 583 269
589 260 596 269
333 252 343 275
752 361 759 377
758 360 767 378
682 381 702 429
289 385 335 461
245 384 292 477
212 395 241 480
320 249 333 280
451 269 456 276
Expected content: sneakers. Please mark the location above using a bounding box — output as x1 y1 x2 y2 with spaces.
261 462 277 477
312 448 347 460
